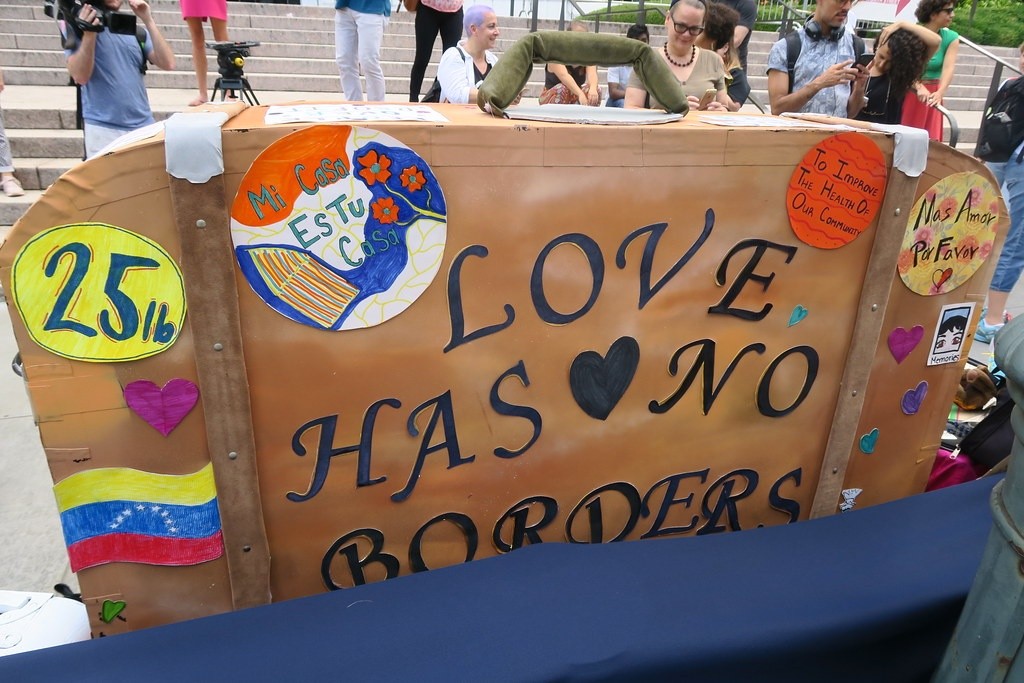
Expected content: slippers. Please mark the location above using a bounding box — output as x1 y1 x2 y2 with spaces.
189 100 205 106
227 91 236 102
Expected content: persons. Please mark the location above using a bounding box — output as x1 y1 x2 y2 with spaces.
538 21 601 108
624 0 757 114
61 0 174 162
765 0 868 118
973 41 1024 344
859 21 942 125
900 0 960 142
0 68 25 197
605 24 650 107
410 0 463 103
180 0 236 105
335 0 391 102
436 5 520 105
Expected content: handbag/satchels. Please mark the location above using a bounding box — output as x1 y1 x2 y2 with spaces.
940 359 1016 458
421 48 466 102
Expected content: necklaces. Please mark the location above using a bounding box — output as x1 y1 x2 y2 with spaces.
663 42 695 66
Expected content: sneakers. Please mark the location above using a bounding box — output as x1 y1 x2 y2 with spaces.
979 307 1011 324
973 319 1005 343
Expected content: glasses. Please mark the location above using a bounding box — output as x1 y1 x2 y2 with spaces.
671 12 705 35
941 8 953 14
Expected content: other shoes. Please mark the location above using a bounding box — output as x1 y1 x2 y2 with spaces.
0 176 24 196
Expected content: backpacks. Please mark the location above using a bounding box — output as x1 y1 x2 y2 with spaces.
973 75 1024 163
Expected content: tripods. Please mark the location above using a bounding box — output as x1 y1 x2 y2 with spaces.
207 42 260 106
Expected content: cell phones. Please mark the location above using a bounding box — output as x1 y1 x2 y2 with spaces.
850 53 876 72
698 89 717 110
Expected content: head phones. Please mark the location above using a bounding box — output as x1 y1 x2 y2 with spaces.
803 13 845 41
